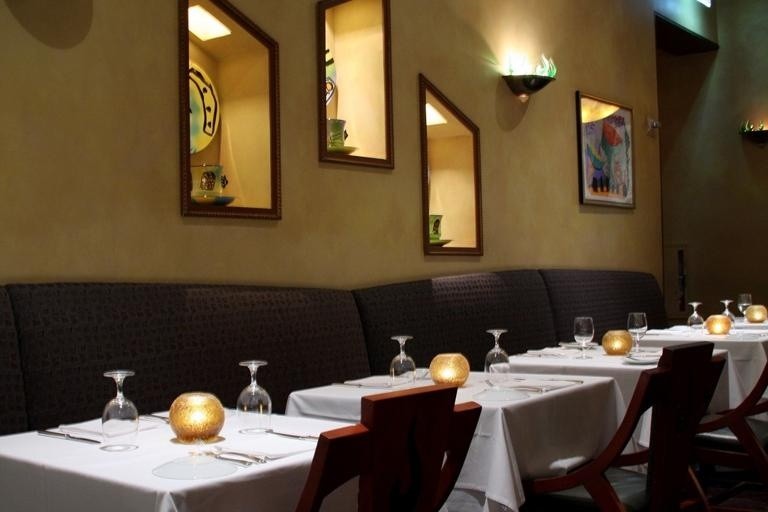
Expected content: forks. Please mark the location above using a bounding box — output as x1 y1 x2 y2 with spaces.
144 414 171 423
208 444 266 465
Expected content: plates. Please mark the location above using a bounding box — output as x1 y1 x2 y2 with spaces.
328 146 358 155
431 240 452 247
192 194 237 206
623 355 661 365
189 60 220 155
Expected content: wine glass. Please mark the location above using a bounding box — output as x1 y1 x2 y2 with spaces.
574 317 595 360
391 335 417 385
688 292 754 331
103 370 137 447
626 312 648 357
236 360 270 433
485 328 513 383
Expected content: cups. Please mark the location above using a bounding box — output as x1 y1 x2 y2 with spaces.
327 119 348 146
430 215 444 240
200 164 228 195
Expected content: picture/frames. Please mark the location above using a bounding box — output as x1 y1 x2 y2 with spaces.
576 91 635 209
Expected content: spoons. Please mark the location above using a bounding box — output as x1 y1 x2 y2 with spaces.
483 379 543 394
191 448 252 470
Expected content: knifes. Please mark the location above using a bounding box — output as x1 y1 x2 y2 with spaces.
263 430 321 442
34 428 102 446
